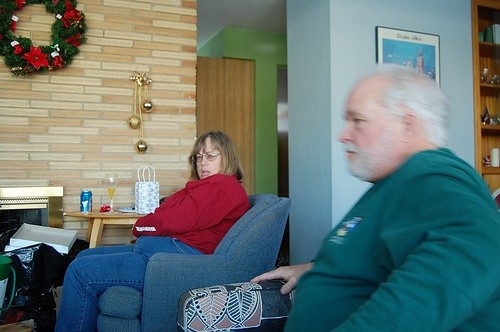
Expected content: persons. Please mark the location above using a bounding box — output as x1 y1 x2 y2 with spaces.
55 130 250 332
251 63 500 332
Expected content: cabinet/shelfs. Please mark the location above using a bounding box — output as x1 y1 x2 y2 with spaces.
471 0 500 193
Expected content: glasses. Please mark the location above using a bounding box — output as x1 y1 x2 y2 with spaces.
196 151 222 161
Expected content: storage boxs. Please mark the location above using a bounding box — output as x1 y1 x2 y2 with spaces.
9 223 79 254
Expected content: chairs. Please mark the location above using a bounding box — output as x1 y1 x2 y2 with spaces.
178 279 292 332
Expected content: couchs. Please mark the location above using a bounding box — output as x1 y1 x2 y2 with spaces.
97 195 292 332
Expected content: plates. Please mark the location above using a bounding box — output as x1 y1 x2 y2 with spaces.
117 206 135 212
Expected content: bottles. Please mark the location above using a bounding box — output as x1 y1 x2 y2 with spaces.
480 106 490 124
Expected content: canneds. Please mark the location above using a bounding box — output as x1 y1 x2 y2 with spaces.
80 190 92 212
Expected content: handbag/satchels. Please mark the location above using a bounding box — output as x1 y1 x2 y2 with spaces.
0 228 42 314
135 166 159 214
27 239 90 332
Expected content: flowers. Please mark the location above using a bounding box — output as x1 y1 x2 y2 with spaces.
0 0 88 78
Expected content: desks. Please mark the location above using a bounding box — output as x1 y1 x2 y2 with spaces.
64 211 145 248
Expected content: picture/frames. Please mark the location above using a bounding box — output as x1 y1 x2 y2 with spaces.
376 26 440 91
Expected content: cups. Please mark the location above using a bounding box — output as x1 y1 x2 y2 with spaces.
490 148 500 167
480 66 489 82
0 257 16 318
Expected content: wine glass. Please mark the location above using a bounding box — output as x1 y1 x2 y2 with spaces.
105 173 118 213
